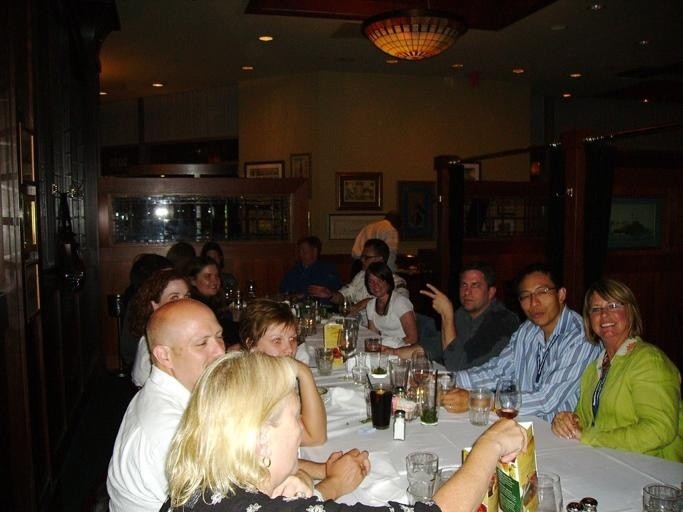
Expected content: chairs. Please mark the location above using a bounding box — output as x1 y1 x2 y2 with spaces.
416 313 436 338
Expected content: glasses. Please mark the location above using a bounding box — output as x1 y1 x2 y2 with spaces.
589 303 622 314
518 287 556 301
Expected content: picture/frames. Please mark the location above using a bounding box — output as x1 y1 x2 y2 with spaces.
20 193 36 252
243 160 284 179
398 180 436 240
610 196 662 249
21 259 41 324
461 159 482 181
289 153 312 178
18 124 34 184
329 214 388 241
335 173 383 210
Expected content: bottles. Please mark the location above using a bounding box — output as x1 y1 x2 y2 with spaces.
392 409 406 441
221 281 359 364
522 470 563 511
565 496 597 511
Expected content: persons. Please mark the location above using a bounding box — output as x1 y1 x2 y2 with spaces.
103 353 528 512
106 243 327 512
282 234 683 463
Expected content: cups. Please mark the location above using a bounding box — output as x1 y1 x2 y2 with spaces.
467 388 495 426
315 337 459 428
404 452 460 512
641 480 683 512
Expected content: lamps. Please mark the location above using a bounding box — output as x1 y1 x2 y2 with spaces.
363 12 466 59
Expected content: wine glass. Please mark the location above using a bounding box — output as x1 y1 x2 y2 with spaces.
494 378 523 418
